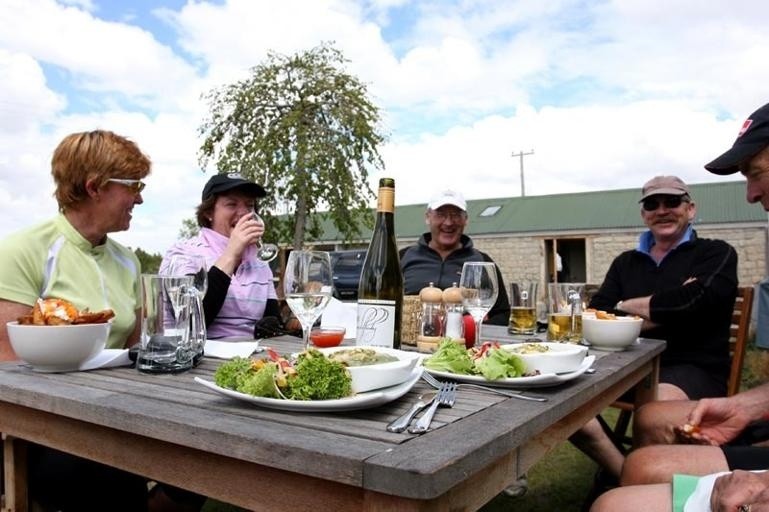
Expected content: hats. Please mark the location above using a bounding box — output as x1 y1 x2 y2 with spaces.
639 176 692 203
202 173 267 199
427 190 466 211
704 103 769 175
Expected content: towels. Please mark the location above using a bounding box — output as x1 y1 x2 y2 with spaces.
199 337 265 360
81 348 134 374
318 295 358 339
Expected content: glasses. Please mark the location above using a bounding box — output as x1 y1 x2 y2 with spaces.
101 177 146 192
643 195 689 211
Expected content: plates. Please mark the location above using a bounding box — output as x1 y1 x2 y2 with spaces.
192 361 428 412
423 352 598 389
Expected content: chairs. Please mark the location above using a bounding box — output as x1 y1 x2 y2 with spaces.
591 286 755 495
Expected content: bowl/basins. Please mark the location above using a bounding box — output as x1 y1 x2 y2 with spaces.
582 314 644 352
5 319 112 374
296 347 422 395
498 343 590 376
309 325 346 346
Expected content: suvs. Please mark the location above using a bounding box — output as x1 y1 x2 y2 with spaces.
309 251 367 302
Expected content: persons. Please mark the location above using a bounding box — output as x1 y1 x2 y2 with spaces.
0 124 154 362
590 102 769 485
400 187 510 327
568 174 741 480
588 470 769 512
158 172 286 340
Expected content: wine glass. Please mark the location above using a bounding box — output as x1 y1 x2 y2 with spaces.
460 261 500 346
283 250 335 350
236 208 279 262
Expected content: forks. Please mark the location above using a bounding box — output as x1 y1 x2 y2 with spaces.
409 381 463 438
420 369 551 406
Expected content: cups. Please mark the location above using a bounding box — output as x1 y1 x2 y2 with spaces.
545 282 586 342
507 282 540 334
129 244 208 373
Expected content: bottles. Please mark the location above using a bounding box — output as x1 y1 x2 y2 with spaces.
415 280 480 351
355 176 405 351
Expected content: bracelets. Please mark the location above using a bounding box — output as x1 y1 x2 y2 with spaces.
616 297 623 311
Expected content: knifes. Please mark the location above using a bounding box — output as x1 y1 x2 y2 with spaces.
385 390 436 432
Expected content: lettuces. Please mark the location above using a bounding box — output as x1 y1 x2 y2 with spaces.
422 337 525 382
